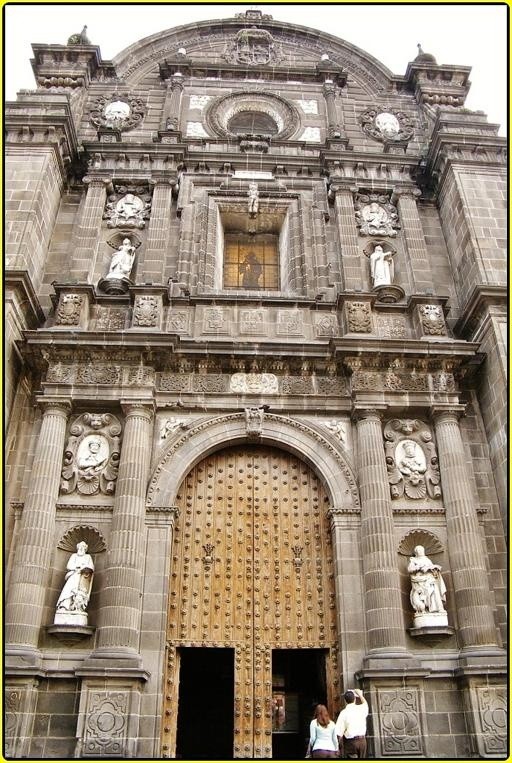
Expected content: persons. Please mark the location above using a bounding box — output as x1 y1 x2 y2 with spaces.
326 417 346 441
54 538 94 611
303 703 339 759
240 250 263 290
116 193 142 217
408 544 448 613
159 415 181 437
368 243 396 288
77 436 107 478
335 687 370 758
364 201 387 226
397 442 428 475
246 180 261 212
105 235 137 280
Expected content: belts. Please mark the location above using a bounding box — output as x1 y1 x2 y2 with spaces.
354 736 364 739
